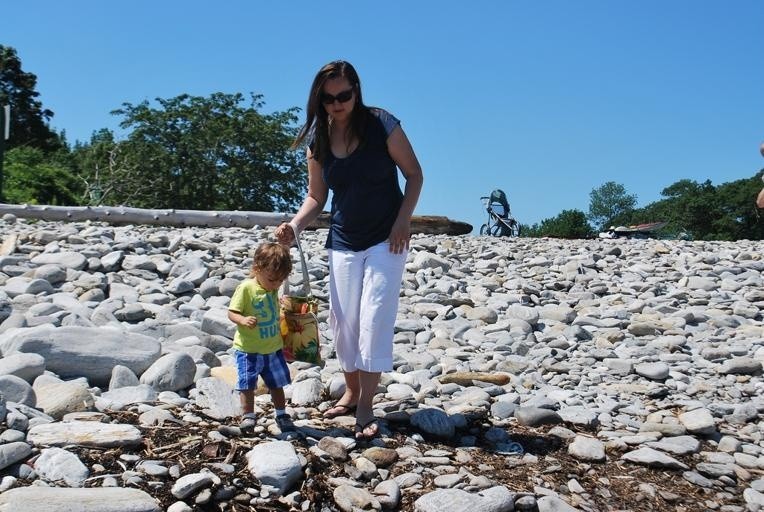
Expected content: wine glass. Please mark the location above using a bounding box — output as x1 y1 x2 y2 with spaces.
389 243 393 246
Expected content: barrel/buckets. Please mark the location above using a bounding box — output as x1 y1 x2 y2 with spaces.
355 419 380 440
322 404 356 419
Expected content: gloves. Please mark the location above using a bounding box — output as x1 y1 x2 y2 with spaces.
606 218 671 236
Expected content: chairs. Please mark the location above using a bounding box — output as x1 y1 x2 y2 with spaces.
238 413 257 431
273 414 297 432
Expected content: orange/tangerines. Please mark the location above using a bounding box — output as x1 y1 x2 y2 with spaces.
479 189 522 237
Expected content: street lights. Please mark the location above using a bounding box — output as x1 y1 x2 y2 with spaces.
279 292 326 369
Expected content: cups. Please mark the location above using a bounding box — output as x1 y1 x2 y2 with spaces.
321 87 355 104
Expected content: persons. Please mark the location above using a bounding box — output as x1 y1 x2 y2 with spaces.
228 241 293 433
275 60 423 440
756 144 764 208
